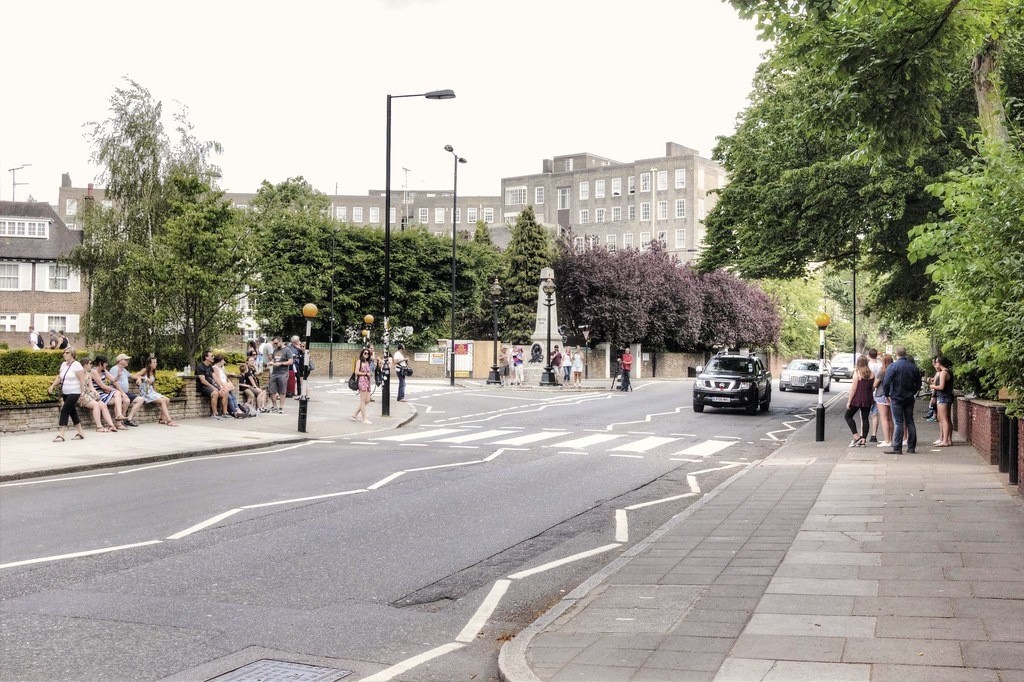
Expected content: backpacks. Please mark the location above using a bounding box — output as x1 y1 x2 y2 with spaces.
32 330 44 348
60 336 68 349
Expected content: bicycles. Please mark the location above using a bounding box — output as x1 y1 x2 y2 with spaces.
352 361 379 396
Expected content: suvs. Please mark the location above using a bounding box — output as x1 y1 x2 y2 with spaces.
830 351 864 382
692 349 773 415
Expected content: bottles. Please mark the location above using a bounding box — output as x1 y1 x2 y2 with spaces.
187 365 191 376
183 366 187 376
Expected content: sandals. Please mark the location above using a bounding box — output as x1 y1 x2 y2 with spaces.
159 418 167 424
96 414 139 432
166 421 179 428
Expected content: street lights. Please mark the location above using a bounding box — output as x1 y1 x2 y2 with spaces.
296 302 319 432
441 143 468 387
361 329 368 349
813 312 831 442
823 280 854 364
486 276 503 386
146 170 224 357
380 88 458 418
328 228 343 379
539 272 558 386
363 313 374 349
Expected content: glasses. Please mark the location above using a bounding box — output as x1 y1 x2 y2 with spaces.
274 341 280 346
362 352 370 355
300 346 305 348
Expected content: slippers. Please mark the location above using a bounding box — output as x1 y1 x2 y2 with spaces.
72 433 87 440
53 436 66 442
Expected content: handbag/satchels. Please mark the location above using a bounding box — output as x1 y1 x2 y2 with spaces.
223 379 234 392
399 367 413 377
374 368 382 387
309 357 315 370
58 387 62 400
349 373 358 391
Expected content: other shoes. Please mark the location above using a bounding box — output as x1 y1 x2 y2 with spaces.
933 438 953 447
210 406 284 419
849 435 915 454
363 419 372 425
350 415 357 421
927 416 936 422
923 415 929 418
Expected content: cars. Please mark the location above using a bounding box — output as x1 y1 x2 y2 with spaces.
779 359 831 393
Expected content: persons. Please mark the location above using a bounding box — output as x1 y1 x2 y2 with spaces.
239 335 314 413
873 345 921 454
572 344 584 387
561 346 573 385
136 357 178 426
351 343 393 422
844 354 875 447
499 344 524 387
860 348 881 443
48 329 71 349
922 356 955 446
28 326 41 348
108 354 144 427
617 348 633 392
76 358 116 432
394 343 409 402
550 345 562 385
48 346 86 442
91 356 130 430
196 351 247 420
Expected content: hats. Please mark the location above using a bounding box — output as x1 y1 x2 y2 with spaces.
116 354 132 362
